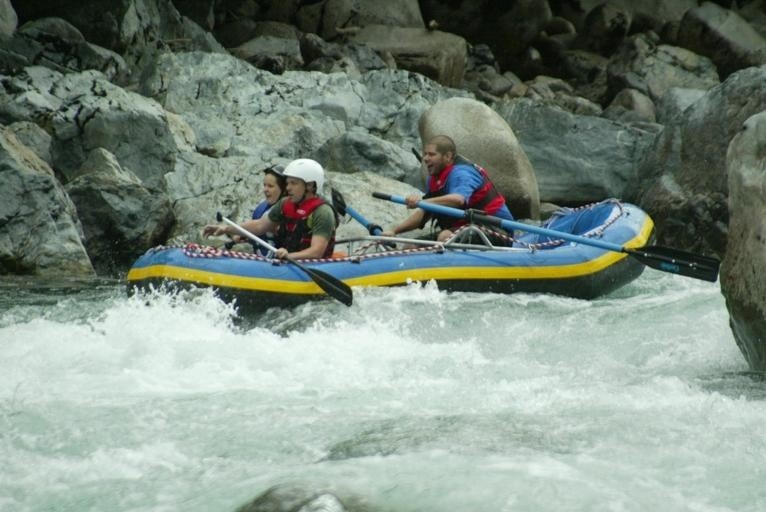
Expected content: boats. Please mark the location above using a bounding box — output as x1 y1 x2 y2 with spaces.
125 199 658 325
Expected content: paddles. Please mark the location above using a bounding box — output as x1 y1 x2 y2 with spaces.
216 211 352 306
331 188 396 249
372 193 719 282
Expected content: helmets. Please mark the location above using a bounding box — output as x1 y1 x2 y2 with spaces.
263 164 286 179
283 159 325 194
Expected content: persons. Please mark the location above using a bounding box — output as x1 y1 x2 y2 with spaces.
202 158 336 259
381 135 514 247
233 162 287 256
